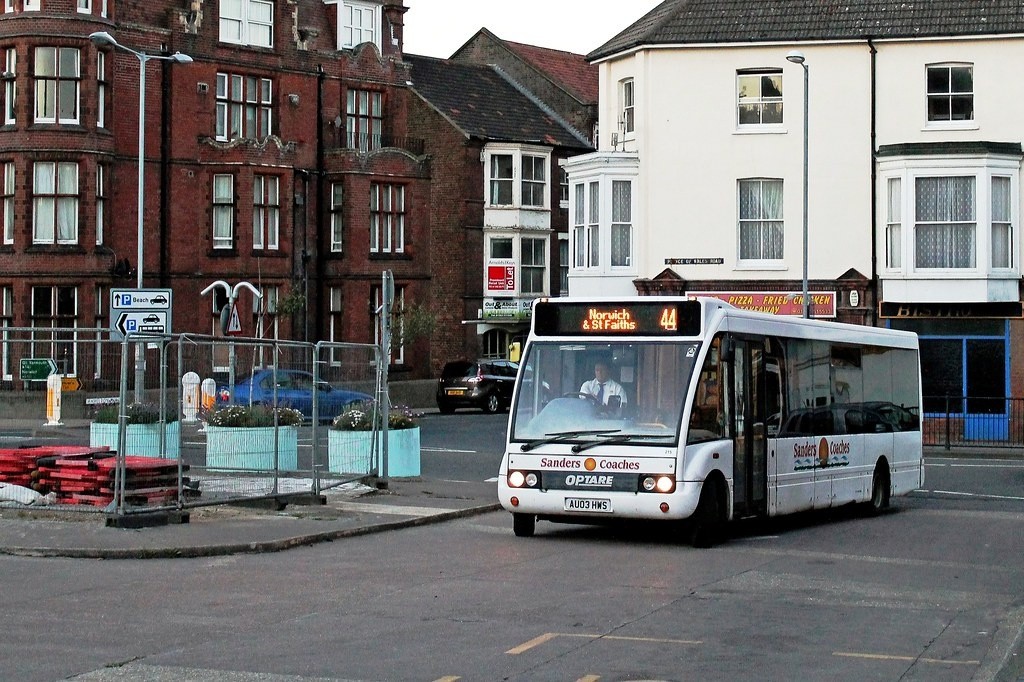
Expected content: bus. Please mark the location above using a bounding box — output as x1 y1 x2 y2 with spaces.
498 295 926 549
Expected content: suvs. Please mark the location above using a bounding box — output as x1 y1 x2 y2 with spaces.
436 356 551 415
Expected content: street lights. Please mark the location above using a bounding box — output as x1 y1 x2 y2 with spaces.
786 49 809 320
89 30 194 396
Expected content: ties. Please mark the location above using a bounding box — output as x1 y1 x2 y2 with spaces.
594 383 607 405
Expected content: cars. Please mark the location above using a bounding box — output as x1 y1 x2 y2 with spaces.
215 367 375 423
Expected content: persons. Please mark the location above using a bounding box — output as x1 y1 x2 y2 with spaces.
579 358 627 409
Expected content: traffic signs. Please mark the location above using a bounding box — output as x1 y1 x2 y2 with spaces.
20 359 56 381
